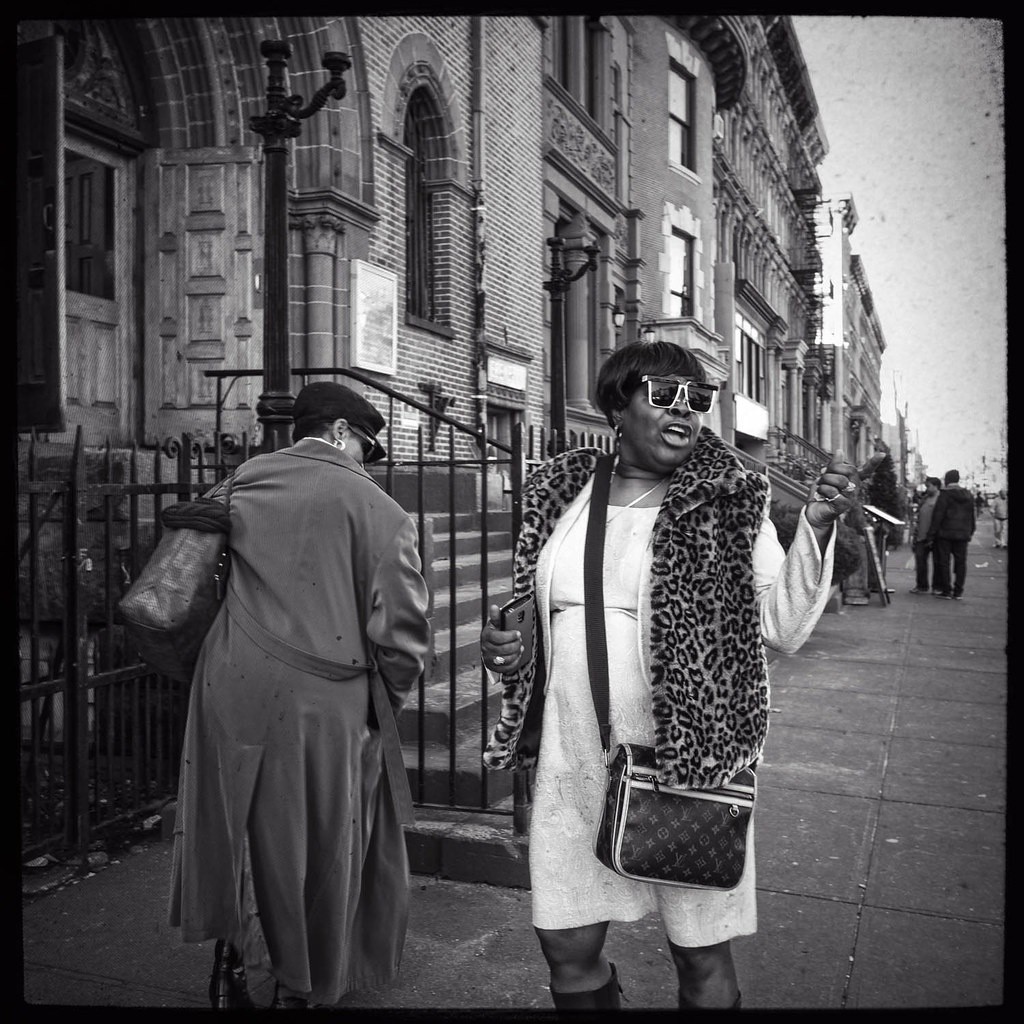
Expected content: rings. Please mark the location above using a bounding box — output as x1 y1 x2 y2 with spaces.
493 657 506 666
843 482 856 494
826 494 841 503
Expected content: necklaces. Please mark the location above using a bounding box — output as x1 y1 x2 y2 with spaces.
605 462 671 529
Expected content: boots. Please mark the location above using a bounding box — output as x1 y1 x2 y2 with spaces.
547 961 623 1009
210 938 242 1008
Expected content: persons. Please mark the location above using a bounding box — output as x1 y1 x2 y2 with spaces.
479 343 856 1007
907 470 976 600
976 491 991 521
157 382 433 1008
989 489 1008 549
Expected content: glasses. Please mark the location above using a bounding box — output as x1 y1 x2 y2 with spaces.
327 423 376 463
637 374 719 414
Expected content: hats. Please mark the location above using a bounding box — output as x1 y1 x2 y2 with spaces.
293 382 387 463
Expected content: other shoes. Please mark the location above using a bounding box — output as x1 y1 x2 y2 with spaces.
996 545 1000 548
952 593 962 599
910 586 928 592
1003 545 1008 550
936 592 951 600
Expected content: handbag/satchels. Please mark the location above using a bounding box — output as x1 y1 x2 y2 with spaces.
118 470 232 632
595 743 756 891
906 554 917 570
